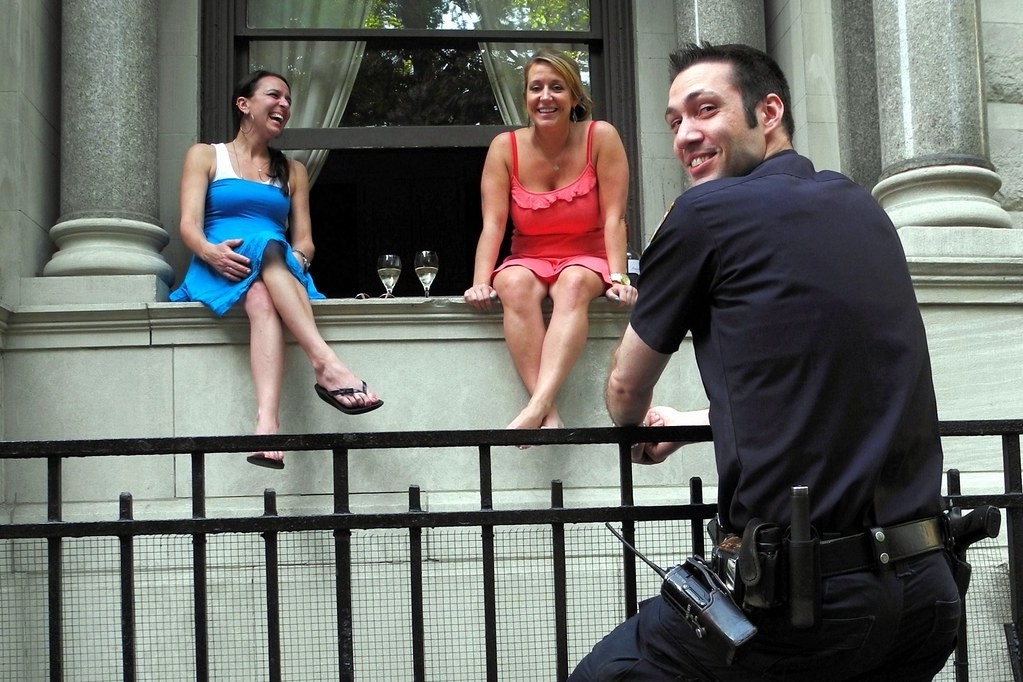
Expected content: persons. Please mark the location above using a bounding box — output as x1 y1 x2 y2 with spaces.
168 70 384 469
464 48 638 450
564 39 962 682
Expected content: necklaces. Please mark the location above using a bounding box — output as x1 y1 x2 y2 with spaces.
233 139 270 178
534 123 570 172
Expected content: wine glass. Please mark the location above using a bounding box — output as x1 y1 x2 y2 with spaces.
376 254 401 297
414 250 439 298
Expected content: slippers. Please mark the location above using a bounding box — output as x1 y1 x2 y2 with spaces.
315 381 384 415
247 453 285 470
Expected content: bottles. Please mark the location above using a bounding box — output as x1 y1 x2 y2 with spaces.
625 220 640 288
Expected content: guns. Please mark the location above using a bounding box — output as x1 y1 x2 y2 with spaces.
936 500 1001 602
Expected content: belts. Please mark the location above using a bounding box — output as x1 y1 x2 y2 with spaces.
785 516 949 581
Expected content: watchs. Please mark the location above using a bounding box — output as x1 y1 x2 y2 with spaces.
610 273 630 286
292 250 310 275
